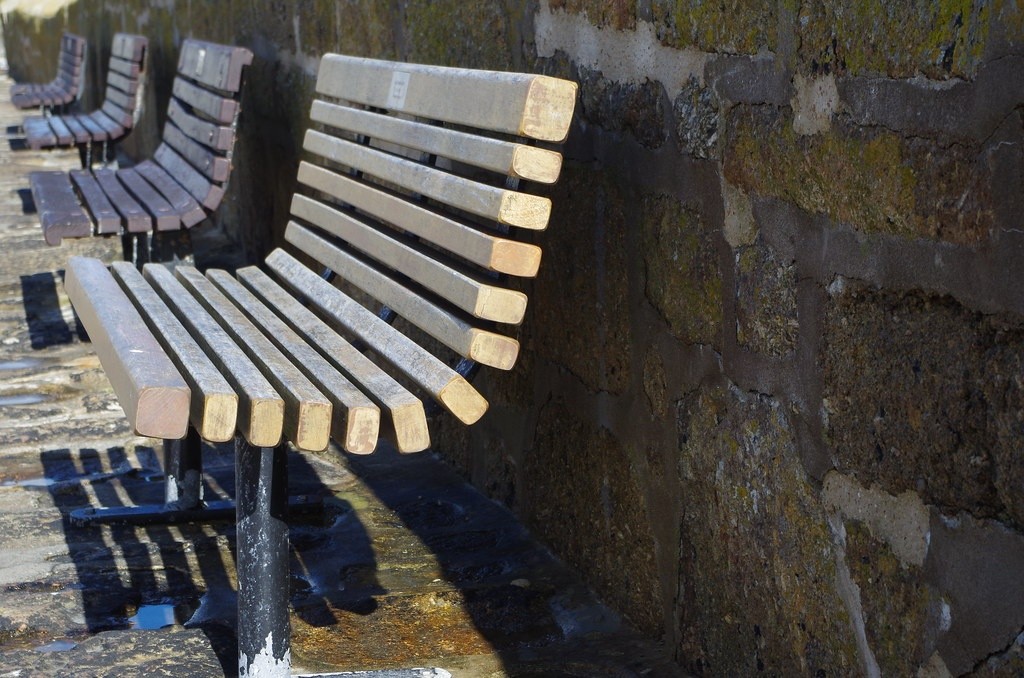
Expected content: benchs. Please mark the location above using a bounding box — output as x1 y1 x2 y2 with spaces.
10 34 89 151
66 49 580 678
27 35 256 270
21 31 152 172
